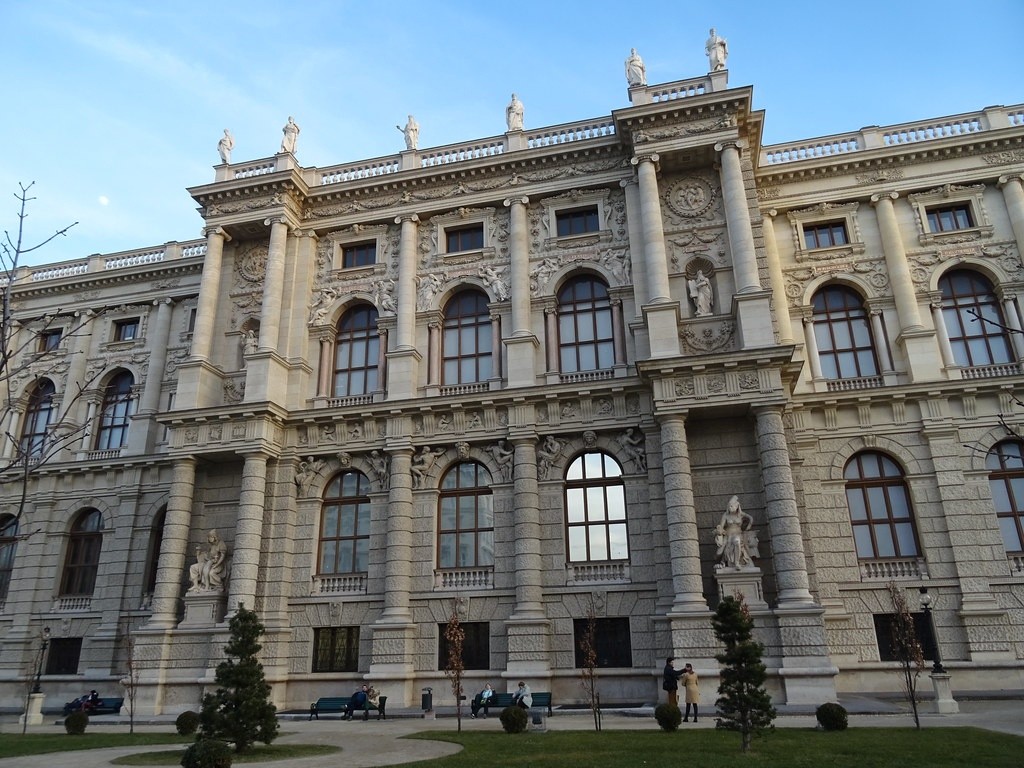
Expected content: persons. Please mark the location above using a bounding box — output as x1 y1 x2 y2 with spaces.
663 657 688 706
294 399 649 501
340 685 368 721
218 26 731 157
468 683 497 719
305 248 632 310
692 270 714 316
188 529 227 592
713 494 754 571
363 686 380 720
681 663 700 723
80 689 98 713
512 681 533 710
240 329 259 369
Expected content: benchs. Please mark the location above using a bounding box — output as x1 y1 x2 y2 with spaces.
471 692 552 717
309 696 388 720
64 698 124 714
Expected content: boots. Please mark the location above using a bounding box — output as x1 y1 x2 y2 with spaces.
683 715 688 722
693 717 697 722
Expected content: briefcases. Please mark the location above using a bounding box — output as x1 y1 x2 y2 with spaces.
355 692 367 703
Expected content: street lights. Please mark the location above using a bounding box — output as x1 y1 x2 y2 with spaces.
21 627 52 727
918 585 961 717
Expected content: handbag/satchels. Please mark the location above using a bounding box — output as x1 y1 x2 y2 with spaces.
481 698 487 704
511 694 523 705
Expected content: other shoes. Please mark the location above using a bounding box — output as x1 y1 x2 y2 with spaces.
365 715 368 720
468 713 474 719
348 716 352 721
484 714 486 719
